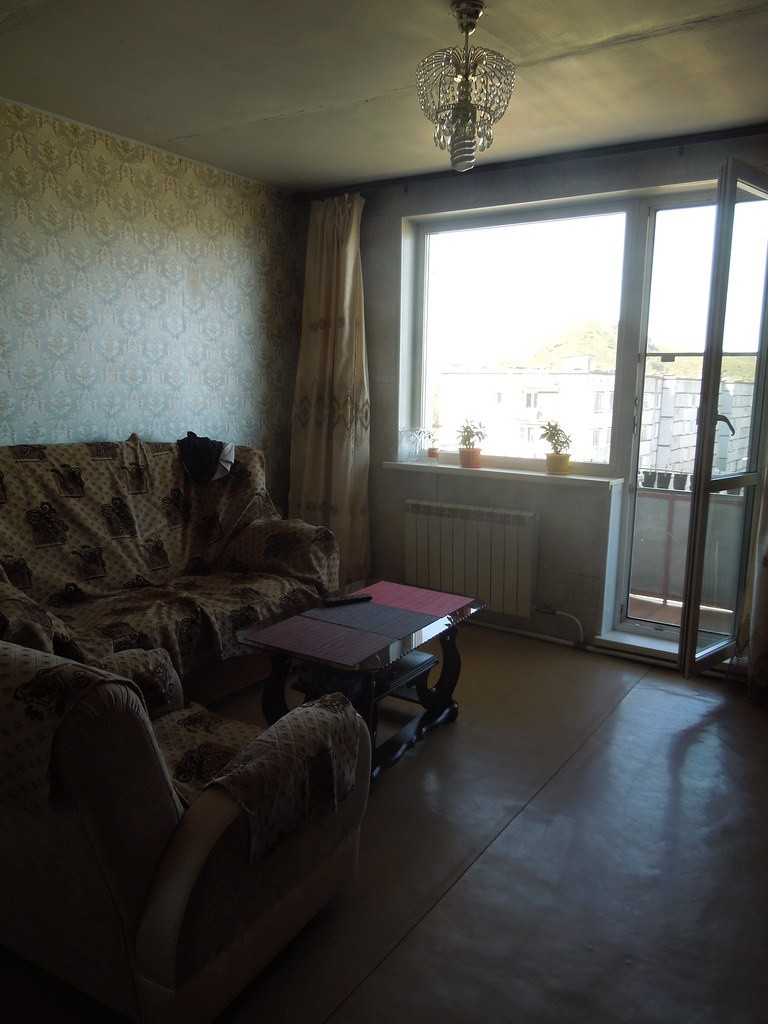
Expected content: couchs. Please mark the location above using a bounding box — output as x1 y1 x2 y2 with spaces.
0 639 371 1023
1 433 341 706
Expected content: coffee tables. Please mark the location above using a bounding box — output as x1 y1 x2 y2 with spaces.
236 575 487 791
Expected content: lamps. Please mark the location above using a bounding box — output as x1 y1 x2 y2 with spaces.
414 0 517 173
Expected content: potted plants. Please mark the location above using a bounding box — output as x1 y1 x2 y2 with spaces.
537 420 573 475
455 415 488 467
419 429 439 462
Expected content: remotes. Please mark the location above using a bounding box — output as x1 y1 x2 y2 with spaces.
324 593 373 608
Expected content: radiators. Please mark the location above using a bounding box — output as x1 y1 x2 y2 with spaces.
405 498 540 619
